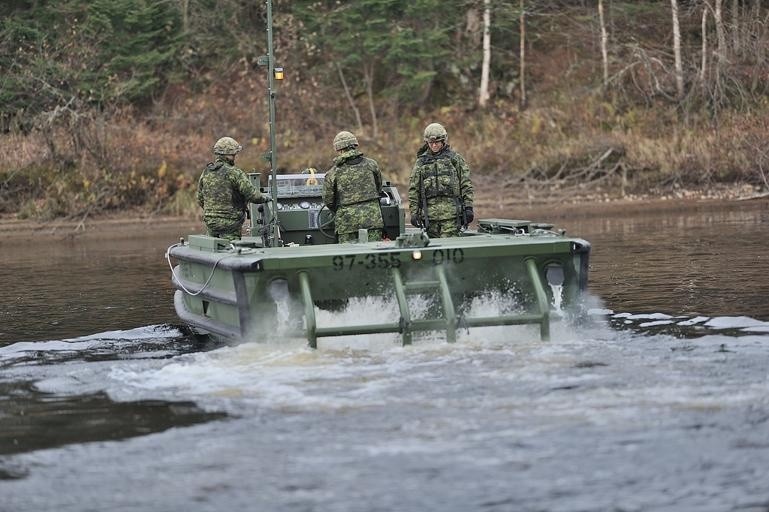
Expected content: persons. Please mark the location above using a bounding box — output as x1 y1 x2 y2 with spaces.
197 137 274 250
409 123 474 239
322 130 384 245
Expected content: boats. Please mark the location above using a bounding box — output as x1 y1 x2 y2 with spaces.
156 168 605 358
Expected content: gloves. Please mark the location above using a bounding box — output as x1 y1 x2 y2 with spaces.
466 206 474 223
410 213 423 228
261 194 274 204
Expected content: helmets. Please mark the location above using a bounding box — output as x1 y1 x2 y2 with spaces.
423 123 449 143
333 130 359 151
213 136 243 155
